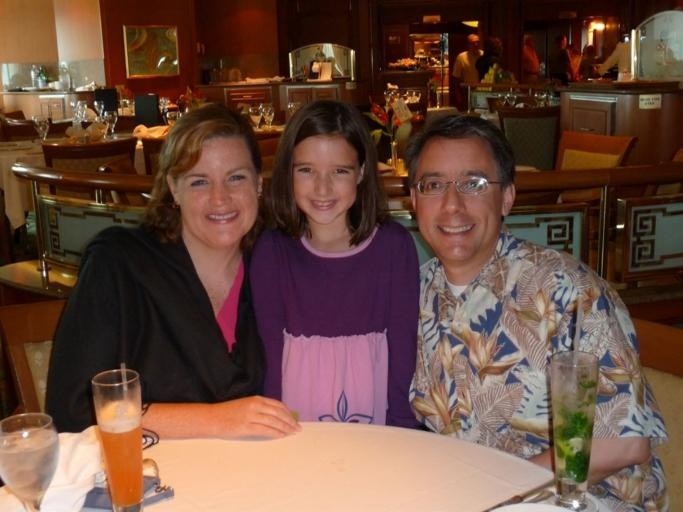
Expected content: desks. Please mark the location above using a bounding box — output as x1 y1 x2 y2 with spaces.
0 420 645 511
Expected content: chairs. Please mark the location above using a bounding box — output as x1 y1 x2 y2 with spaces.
0 298 69 412
632 316 681 511
0 83 682 263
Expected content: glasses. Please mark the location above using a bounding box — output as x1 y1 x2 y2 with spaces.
410 174 505 197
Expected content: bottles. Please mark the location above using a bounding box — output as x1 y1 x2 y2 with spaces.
550 349 597 511
617 69 630 81
385 159 405 176
29 64 47 91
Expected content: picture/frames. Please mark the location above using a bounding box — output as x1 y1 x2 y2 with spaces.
120 23 181 83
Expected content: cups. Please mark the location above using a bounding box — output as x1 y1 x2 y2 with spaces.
308 60 332 80
90 370 149 511
165 112 179 127
53 80 65 91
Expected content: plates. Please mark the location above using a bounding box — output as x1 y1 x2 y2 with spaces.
487 502 572 511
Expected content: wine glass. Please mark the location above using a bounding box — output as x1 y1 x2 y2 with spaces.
248 101 300 129
495 88 553 110
22 101 119 152
0 411 64 512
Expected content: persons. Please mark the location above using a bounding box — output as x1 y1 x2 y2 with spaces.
402 114 669 511
548 35 576 83
520 34 540 85
474 38 504 82
249 98 423 429
452 33 485 109
41 99 304 441
577 44 599 79
